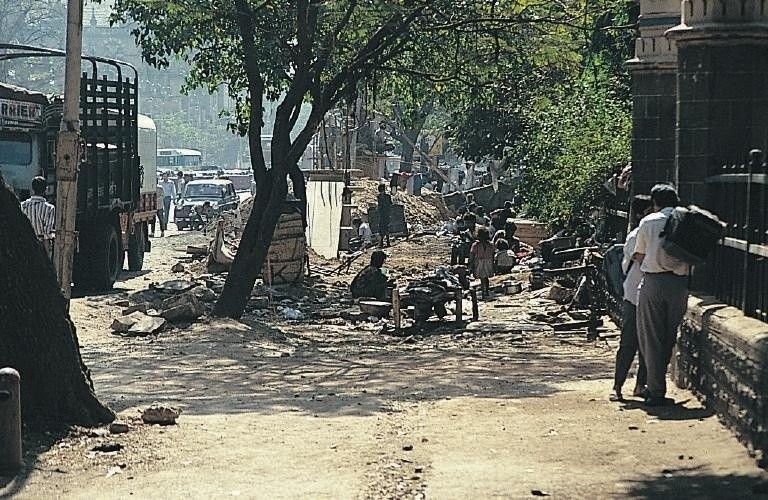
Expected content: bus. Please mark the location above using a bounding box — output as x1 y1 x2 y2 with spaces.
157 149 201 172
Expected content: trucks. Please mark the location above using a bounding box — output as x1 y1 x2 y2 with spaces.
1 43 158 293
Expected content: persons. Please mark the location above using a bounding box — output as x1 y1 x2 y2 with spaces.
158 173 178 231
631 181 694 407
350 250 392 303
373 183 394 250
148 176 166 237
372 120 396 156
18 175 57 264
347 216 374 252
169 170 185 223
417 161 520 301
608 192 656 402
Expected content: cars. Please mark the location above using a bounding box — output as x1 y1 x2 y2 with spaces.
172 180 240 230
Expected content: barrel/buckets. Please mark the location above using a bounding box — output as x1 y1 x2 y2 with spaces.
260 203 307 285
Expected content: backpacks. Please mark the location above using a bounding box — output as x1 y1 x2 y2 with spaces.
658 206 731 266
602 243 634 297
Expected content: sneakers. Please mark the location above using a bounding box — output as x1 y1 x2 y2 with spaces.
609 389 622 401
634 387 666 405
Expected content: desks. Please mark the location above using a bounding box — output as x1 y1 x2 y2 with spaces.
392 288 478 329
